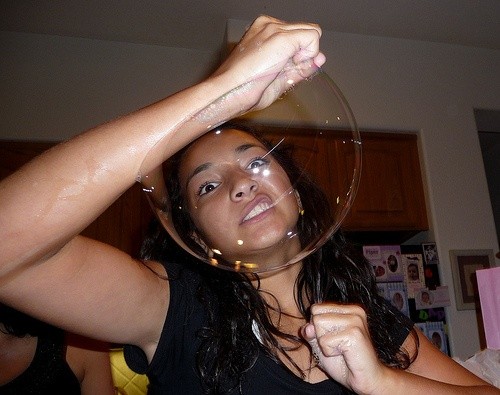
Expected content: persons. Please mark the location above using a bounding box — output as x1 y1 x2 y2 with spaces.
0 14 498 395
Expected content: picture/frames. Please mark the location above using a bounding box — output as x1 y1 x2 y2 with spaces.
449 249 497 312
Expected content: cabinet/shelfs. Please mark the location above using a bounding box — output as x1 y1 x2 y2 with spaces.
243 124 430 245
0 140 158 262
